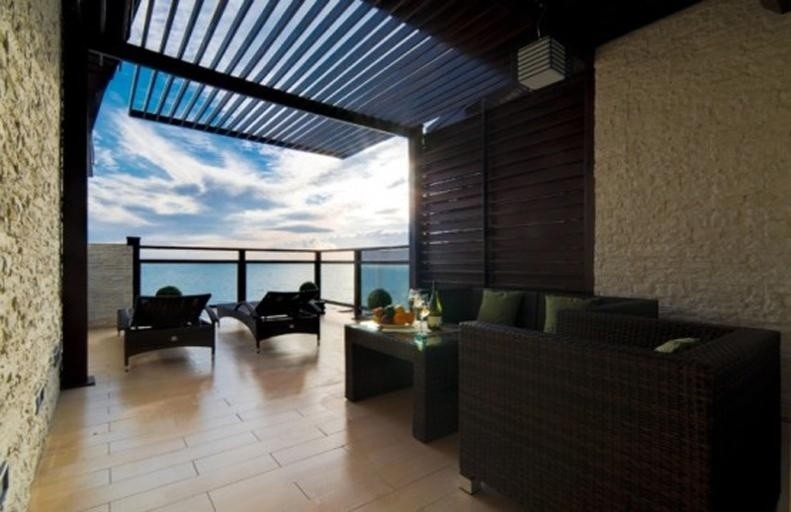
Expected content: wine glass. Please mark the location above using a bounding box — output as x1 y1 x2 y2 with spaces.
413 294 431 337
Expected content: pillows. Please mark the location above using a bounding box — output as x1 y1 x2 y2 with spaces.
478 287 601 336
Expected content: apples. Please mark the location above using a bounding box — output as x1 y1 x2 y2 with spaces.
373 305 413 325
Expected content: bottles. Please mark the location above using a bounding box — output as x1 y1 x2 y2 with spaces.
427 280 443 330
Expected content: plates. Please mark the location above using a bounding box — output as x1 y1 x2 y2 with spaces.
375 324 412 329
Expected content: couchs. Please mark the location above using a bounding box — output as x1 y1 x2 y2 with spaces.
410 285 660 336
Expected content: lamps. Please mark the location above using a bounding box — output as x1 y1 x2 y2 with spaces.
517 1 568 90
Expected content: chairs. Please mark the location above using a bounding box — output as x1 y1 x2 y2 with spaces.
458 308 784 511
117 290 322 372
